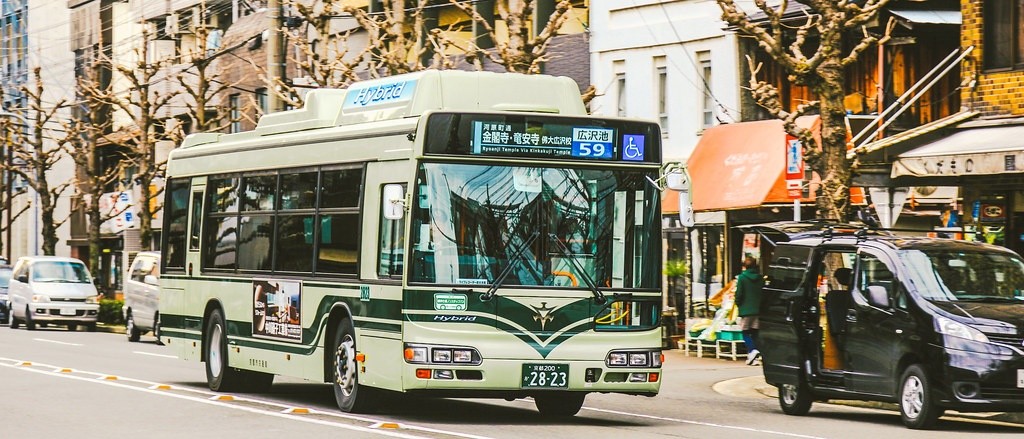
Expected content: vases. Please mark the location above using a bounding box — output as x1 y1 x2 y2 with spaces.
677 342 685 349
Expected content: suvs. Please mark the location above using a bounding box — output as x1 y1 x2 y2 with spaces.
4 254 101 332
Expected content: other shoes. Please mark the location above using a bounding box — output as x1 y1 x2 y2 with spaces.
745 349 760 365
752 358 760 366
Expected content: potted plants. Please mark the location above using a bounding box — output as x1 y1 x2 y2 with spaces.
666 259 684 349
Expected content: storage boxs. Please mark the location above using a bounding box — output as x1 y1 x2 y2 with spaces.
688 328 706 337
714 330 745 340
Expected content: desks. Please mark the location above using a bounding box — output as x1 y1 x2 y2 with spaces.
716 340 748 361
685 337 716 358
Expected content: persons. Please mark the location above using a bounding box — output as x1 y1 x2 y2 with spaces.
734 256 765 366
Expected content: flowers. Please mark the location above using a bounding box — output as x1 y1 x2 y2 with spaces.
678 338 685 343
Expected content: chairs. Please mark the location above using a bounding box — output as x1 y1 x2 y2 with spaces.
824 268 853 337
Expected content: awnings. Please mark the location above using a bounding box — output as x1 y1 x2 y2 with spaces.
661 114 822 214
890 123 1024 178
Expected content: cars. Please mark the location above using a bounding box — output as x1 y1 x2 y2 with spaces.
0 257 14 324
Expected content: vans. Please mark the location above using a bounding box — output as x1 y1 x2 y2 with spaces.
119 250 163 342
757 213 1024 430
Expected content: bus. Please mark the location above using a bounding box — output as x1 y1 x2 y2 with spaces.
158 66 696 419
